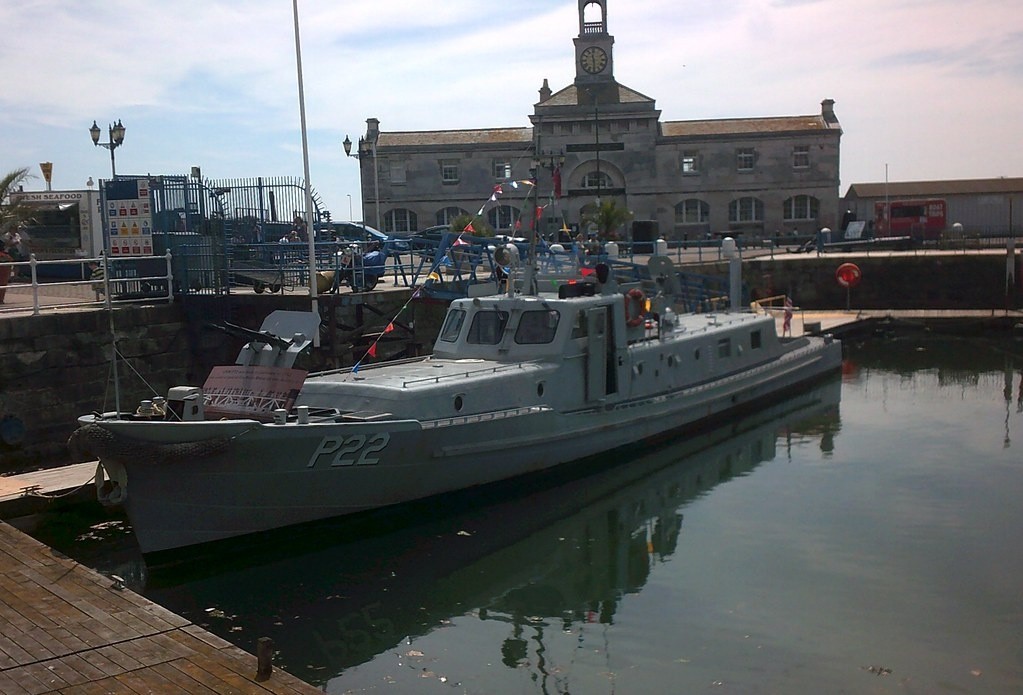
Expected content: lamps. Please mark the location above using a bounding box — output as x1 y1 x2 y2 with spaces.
87 177 94 190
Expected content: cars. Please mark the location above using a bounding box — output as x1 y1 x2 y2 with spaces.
329 220 408 253
408 223 508 252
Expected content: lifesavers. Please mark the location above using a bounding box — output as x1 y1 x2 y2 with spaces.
835 262 861 288
624 288 646 327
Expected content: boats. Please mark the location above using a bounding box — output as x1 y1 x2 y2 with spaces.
108 370 843 685
75 0 844 552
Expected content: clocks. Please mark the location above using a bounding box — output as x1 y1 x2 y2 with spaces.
580 46 607 74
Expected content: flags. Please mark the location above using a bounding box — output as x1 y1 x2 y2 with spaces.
552 169 562 199
783 294 793 331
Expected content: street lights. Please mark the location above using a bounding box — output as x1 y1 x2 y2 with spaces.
343 134 373 241
90 119 129 182
347 194 354 222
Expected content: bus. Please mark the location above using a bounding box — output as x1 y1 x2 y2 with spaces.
873 198 947 239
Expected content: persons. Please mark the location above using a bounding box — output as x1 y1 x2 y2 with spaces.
575 233 586 265
230 230 245 245
87 261 112 302
793 228 800 236
0 224 30 304
817 225 825 257
279 234 289 243
586 234 601 255
289 231 301 243
294 216 308 243
776 230 780 248
245 220 266 243
657 232 667 241
